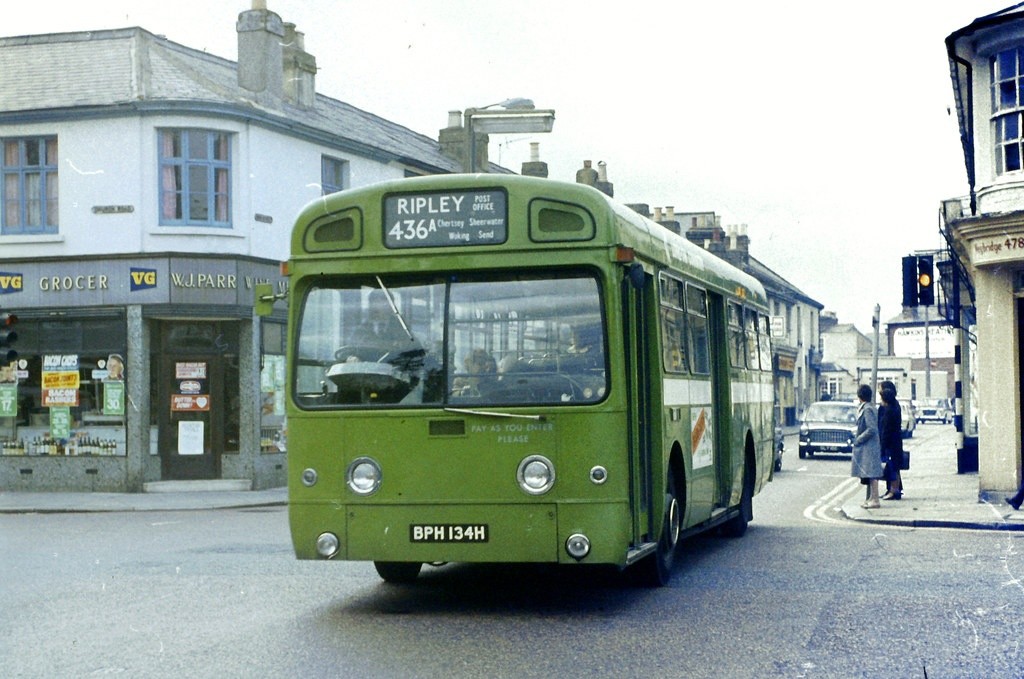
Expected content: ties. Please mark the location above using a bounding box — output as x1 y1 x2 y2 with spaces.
378 322 385 336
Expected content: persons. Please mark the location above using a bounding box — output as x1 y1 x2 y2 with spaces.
876 379 903 500
346 287 421 354
850 384 883 510
452 345 508 397
820 389 831 401
566 329 594 354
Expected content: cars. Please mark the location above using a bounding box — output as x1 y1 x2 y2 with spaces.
772 425 784 472
915 396 953 424
896 397 916 438
798 401 860 459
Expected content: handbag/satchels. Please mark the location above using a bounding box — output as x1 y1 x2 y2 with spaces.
899 451 910 469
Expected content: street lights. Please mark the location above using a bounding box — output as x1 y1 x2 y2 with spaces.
462 95 537 172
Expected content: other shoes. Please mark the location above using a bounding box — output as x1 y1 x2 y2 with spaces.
883 493 901 500
879 490 889 497
862 501 880 507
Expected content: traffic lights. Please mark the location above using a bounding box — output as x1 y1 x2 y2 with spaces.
0 307 18 366
917 255 934 305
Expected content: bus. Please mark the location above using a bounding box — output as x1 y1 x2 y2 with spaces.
254 173 776 588
